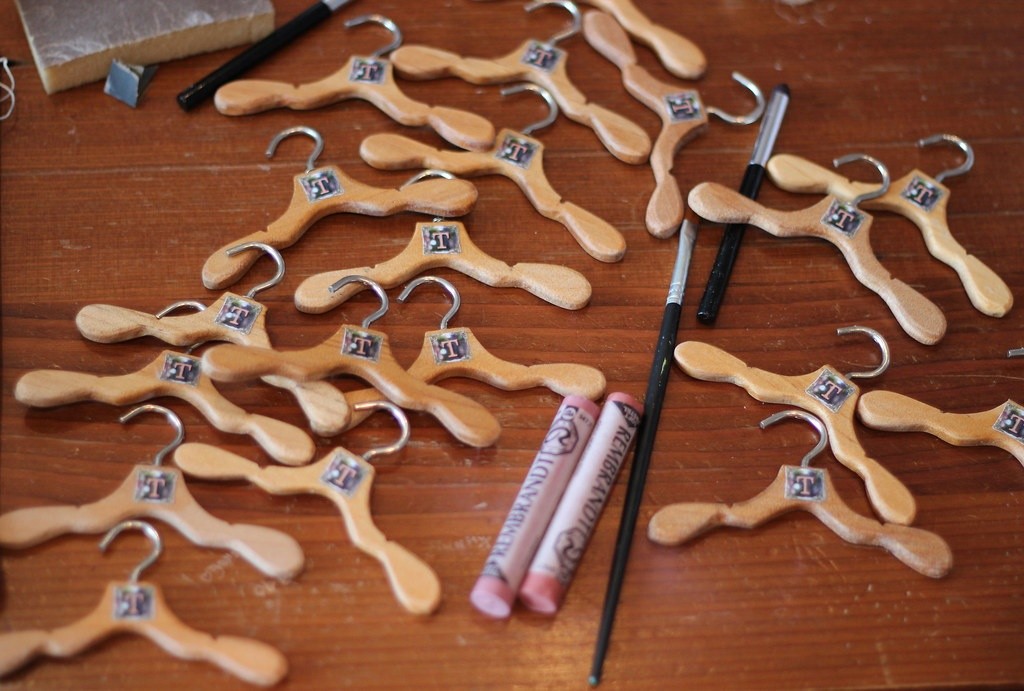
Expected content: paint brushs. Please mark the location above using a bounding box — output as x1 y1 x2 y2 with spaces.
586 204 702 687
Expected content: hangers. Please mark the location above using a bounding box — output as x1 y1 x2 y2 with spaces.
1 0 1024 691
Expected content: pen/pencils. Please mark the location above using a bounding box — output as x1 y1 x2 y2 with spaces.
695 83 792 326
174 0 352 113
